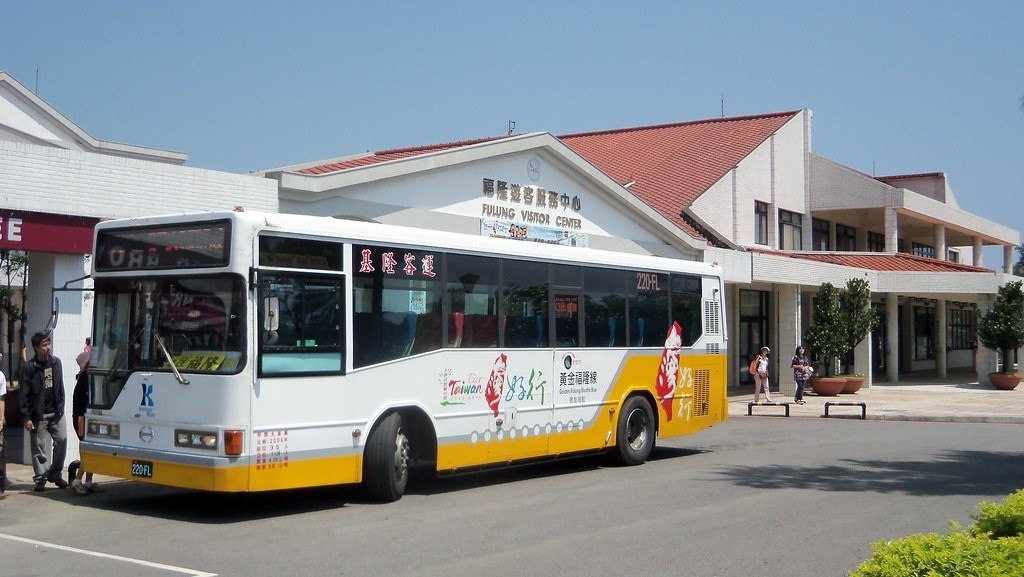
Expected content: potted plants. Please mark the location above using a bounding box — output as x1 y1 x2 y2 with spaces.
975 279 1024 389
803 277 881 396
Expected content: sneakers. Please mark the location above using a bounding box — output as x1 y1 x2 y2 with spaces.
85 483 104 492
70 482 86 494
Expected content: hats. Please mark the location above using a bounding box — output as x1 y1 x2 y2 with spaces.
76 352 90 372
761 347 770 351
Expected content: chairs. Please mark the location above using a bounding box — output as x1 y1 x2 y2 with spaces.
353 309 700 367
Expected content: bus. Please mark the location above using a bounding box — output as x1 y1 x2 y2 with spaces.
52 210 727 500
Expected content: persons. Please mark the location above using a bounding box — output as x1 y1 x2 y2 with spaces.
0 350 21 500
791 346 810 404
753 347 772 402
21 332 67 491
84 337 91 352
72 352 104 494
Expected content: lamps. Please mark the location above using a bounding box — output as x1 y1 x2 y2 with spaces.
945 300 977 307
899 295 903 300
881 297 885 303
909 296 937 303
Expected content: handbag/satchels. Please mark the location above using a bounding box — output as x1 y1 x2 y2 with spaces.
756 370 768 379
749 355 760 374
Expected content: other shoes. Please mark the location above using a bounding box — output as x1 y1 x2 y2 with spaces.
794 397 806 405
47 473 68 489
35 483 45 491
